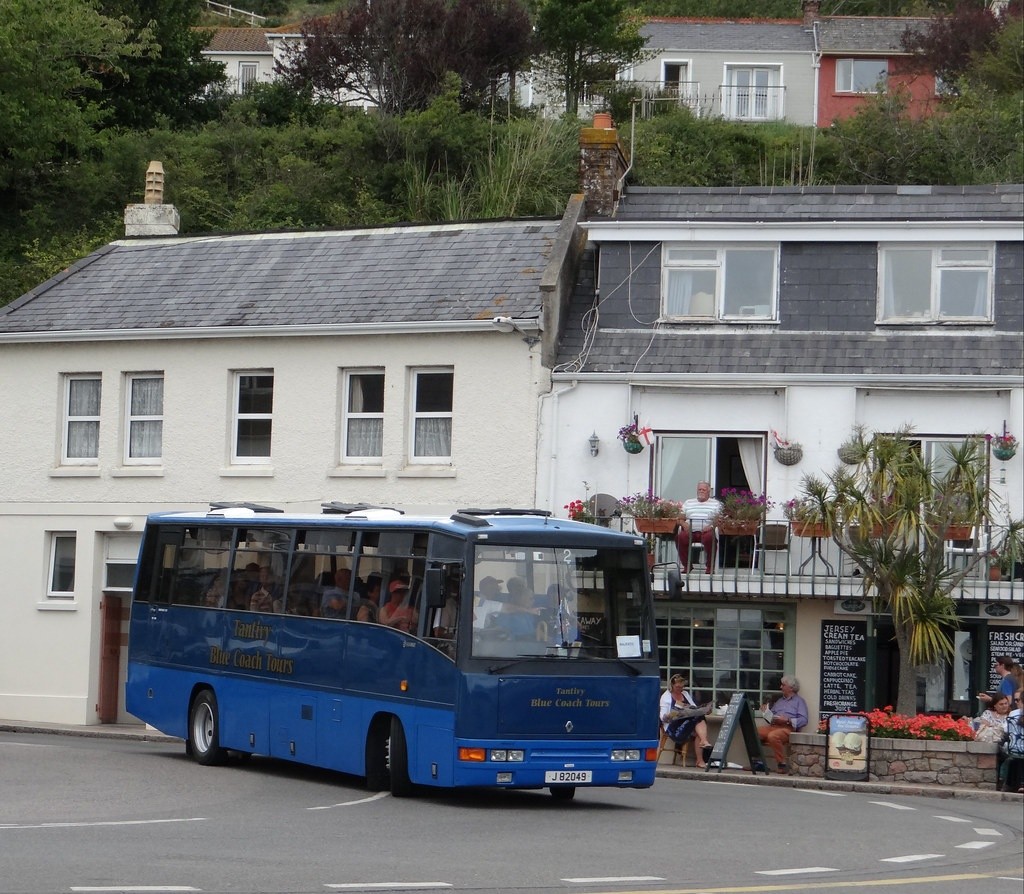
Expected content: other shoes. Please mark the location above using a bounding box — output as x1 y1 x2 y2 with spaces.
745 767 752 771
1018 782 1024 792
681 566 694 574
705 568 715 575
779 764 787 774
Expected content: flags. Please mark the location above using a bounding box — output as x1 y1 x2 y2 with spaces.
639 427 655 446
772 431 790 448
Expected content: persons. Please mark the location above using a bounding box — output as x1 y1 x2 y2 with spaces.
756 676 808 774
206 562 585 654
976 656 1024 794
676 480 724 574
659 673 711 769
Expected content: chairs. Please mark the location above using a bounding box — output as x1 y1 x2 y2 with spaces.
750 520 793 582
655 723 688 768
946 537 986 581
999 715 1024 794
690 528 719 571
760 726 800 769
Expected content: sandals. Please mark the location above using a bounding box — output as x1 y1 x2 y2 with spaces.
699 740 712 749
696 760 707 768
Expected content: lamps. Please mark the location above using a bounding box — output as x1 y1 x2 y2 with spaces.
492 317 536 346
588 429 599 457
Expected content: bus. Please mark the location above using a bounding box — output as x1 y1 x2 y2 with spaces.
124 500 685 804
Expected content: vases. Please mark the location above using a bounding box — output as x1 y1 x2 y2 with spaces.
868 519 912 540
927 522 974 541
985 566 1002 581
715 519 759 535
991 448 1016 460
634 516 677 534
791 519 831 538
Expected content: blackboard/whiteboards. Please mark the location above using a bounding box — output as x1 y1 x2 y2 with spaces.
820 619 868 721
986 625 1024 694
709 691 765 765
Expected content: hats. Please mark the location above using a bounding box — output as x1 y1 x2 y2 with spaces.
479 576 504 590
391 581 409 592
547 584 570 595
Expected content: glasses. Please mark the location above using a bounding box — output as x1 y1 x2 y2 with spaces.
781 683 786 687
993 664 999 666
1014 698 1021 702
675 685 685 689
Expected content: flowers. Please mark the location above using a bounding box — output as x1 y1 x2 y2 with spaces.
713 487 776 528
781 498 827 522
984 433 1019 451
565 500 611 527
614 489 684 518
